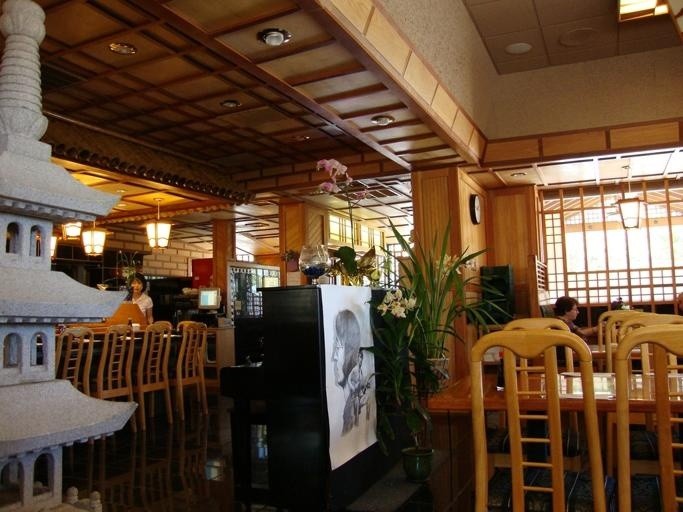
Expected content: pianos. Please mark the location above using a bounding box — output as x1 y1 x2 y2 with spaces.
219 282 410 509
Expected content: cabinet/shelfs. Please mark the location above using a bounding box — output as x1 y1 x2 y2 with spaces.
192 326 236 379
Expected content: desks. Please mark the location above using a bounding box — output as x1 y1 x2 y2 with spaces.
429 371 683 508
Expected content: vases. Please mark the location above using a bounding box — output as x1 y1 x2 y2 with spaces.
330 254 382 286
418 357 449 389
402 446 433 484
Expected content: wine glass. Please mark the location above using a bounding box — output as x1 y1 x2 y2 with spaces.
299 243 332 286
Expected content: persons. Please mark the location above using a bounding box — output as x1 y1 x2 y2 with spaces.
332 310 375 437
126 273 154 324
553 296 606 336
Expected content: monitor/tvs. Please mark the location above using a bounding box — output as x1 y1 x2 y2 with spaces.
196 288 221 313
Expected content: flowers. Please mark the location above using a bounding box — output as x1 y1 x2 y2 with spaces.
372 286 441 450
316 160 385 285
369 212 505 356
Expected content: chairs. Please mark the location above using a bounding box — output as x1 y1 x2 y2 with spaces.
55 321 210 446
467 308 683 512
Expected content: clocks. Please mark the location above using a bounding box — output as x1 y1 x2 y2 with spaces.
470 192 482 223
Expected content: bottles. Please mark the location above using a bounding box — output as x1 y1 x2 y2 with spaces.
496 352 505 391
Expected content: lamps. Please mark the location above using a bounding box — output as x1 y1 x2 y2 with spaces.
146 198 172 249
62 219 107 256
617 165 641 228
